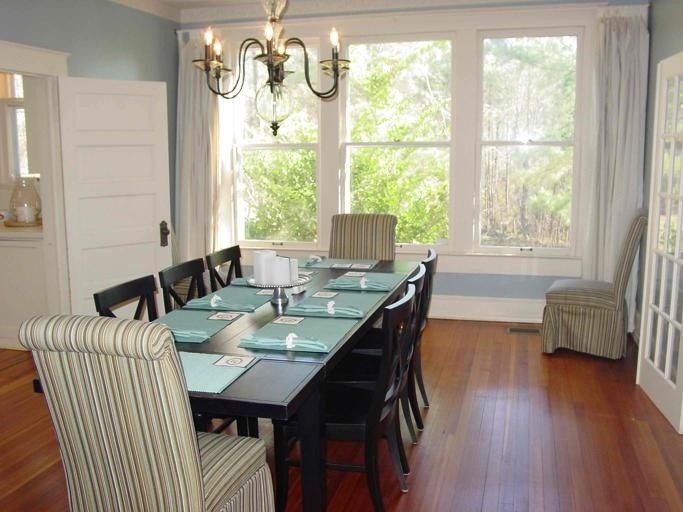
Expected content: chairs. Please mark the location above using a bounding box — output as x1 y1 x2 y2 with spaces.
542 213 646 361
207 245 242 292
321 263 428 476
159 258 208 314
18 312 275 510
274 283 417 512
348 248 437 429
94 274 248 438
328 213 397 258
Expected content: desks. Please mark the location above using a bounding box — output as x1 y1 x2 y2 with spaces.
34 263 421 512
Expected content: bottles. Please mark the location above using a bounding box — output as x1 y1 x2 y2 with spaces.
9 177 41 221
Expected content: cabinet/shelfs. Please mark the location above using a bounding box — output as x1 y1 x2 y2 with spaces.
0 239 44 354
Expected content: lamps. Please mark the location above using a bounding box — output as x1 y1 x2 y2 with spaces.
190 0 351 138
